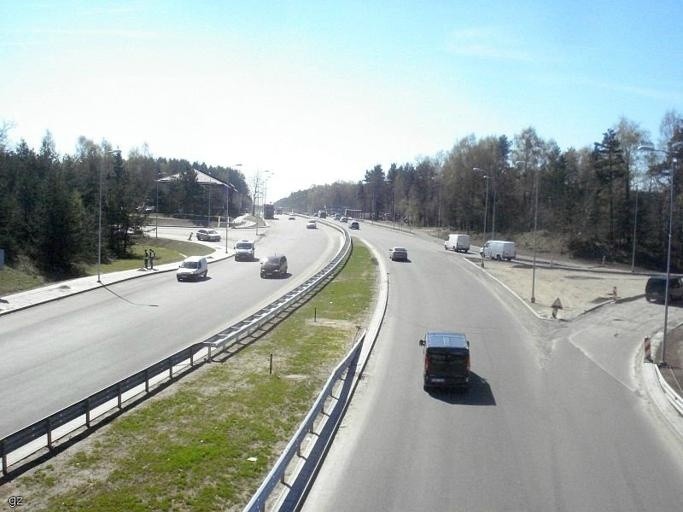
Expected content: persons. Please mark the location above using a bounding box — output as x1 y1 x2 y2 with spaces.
149 248 155 269
144 249 149 268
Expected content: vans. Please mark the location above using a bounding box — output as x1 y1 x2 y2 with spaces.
234 240 255 261
177 256 208 282
646 276 682 304
260 256 287 278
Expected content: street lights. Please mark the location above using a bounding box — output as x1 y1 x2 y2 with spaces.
515 161 540 303
226 164 242 254
474 167 488 247
637 145 675 365
97 148 122 283
255 169 270 234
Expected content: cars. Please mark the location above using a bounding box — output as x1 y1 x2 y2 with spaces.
390 247 407 262
288 214 295 220
352 222 359 229
340 216 346 222
307 220 316 228
196 229 220 242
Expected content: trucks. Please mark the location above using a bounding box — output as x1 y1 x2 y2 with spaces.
418 332 470 390
480 240 515 261
445 233 471 252
317 210 326 218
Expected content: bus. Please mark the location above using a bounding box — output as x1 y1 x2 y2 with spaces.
264 204 274 219
